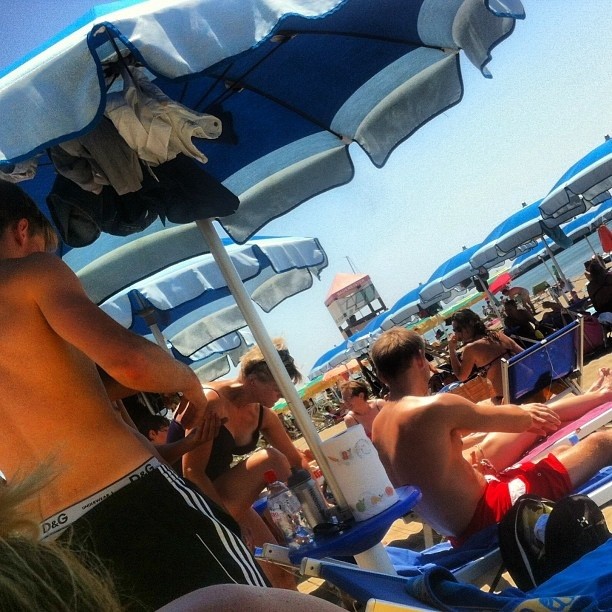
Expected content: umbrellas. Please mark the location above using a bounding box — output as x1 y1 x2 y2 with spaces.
70 234 329 398
557 212 606 272
419 239 538 318
378 283 451 332
138 327 254 416
597 198 611 225
470 188 609 296
345 307 417 358
508 236 570 286
306 343 353 381
537 135 611 229
1 0 526 516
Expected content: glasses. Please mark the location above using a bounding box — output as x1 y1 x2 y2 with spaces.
452 325 465 333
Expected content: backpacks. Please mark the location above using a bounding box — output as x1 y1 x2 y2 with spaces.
498 494 612 592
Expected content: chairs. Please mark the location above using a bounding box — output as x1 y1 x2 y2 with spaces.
500 312 585 404
254 465 612 611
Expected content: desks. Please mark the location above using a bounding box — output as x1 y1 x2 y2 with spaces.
289 485 422 577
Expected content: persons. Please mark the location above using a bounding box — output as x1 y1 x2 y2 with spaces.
147 415 173 447
503 300 540 350
339 379 387 445
3 453 123 612
543 301 562 314
502 289 536 316
461 366 612 477
3 181 276 612
373 329 612 546
174 340 329 590
480 301 493 314
569 289 583 310
448 308 525 448
502 286 536 315
584 256 611 312
324 404 344 420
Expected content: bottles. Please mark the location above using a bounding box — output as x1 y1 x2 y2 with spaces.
263 470 316 549
287 467 335 534
